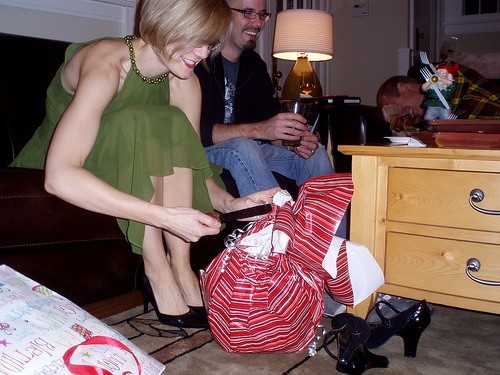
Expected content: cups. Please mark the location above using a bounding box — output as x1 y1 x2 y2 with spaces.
279 100 306 148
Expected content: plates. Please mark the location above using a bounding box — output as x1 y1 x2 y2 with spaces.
384 137 409 143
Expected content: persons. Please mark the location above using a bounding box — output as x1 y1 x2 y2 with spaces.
9 0 288 328
377 68 500 122
193 0 349 315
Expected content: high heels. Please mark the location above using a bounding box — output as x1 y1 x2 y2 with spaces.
139 274 209 329
323 313 389 375
188 305 208 322
365 299 431 358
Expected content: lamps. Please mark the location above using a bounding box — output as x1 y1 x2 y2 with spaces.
272 9 334 99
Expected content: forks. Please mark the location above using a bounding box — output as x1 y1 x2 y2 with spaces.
420 52 444 79
420 66 450 110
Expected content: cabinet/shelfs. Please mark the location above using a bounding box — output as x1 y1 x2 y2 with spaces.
337 145 500 315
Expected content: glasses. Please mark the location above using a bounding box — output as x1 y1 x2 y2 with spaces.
230 7 271 22
207 39 222 51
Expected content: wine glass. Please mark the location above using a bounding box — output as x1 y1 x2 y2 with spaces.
300 72 314 95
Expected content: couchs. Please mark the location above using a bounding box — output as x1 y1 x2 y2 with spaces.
0 32 299 307
